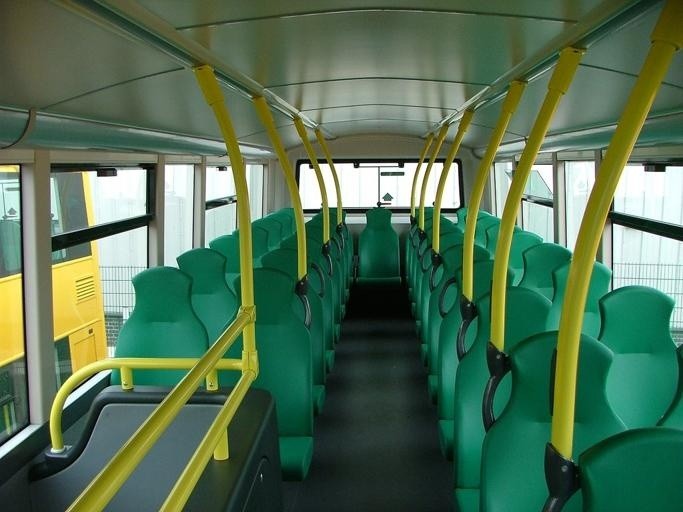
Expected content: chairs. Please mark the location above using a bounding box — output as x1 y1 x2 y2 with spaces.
110 207 352 482
355 210 403 288
406 211 682 508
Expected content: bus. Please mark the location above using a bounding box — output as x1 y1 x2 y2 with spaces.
0 163 110 450
